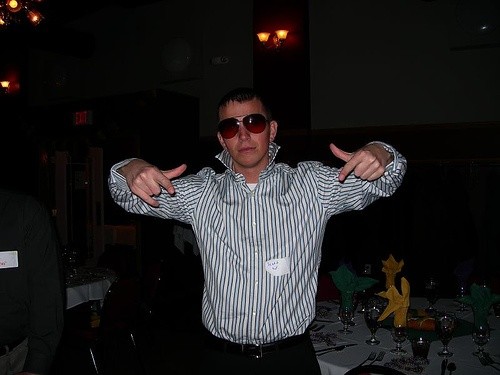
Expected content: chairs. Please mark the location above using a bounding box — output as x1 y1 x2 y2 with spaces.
74 265 159 374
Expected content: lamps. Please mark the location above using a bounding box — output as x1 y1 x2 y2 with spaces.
1 80 11 93
257 28 290 48
0 0 44 27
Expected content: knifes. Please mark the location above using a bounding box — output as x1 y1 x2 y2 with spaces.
316 344 358 353
309 324 325 332
441 359 448 375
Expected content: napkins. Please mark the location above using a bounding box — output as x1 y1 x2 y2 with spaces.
330 266 377 310
459 286 498 323
380 279 409 324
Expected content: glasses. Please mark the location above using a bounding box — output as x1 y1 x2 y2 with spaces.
217 113 270 139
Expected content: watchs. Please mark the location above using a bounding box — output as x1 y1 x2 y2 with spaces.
383 145 393 155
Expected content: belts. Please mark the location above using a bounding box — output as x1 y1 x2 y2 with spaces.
200 323 308 360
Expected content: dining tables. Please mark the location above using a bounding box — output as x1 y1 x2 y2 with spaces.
311 296 500 375
66 265 113 374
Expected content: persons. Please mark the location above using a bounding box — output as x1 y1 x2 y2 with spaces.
108 87 408 375
0 190 66 375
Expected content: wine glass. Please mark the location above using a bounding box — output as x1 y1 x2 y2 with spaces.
435 312 454 357
472 325 489 357
425 277 440 311
454 276 470 311
390 325 408 355
61 249 114 313
364 300 383 345
338 298 357 335
356 265 371 299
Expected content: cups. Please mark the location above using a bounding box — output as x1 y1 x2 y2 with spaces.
411 336 432 359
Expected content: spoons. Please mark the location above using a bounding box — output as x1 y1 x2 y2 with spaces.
317 345 346 357
448 362 456 375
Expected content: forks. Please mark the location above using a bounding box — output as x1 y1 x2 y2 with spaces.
369 352 385 365
479 356 500 372
358 352 376 366
